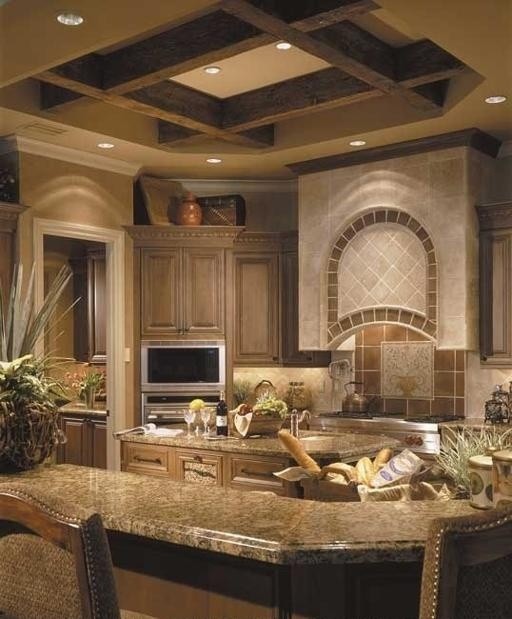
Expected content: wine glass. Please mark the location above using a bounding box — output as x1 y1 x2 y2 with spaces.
181 406 212 439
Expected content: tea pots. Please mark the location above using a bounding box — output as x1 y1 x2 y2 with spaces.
341 379 378 412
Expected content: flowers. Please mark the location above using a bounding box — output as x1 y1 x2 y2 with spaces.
66 366 106 396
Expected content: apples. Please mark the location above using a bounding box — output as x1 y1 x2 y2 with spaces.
189 398 205 412
240 404 253 416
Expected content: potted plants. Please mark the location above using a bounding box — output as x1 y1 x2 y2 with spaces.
0 259 85 474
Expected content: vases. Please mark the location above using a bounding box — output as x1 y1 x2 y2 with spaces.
78 388 106 401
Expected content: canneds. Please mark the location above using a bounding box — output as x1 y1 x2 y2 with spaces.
492 450 512 510
468 455 492 509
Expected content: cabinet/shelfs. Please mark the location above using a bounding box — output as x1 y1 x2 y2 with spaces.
175 447 222 486
58 414 106 468
140 247 225 335
120 441 176 479
230 253 279 364
283 253 314 364
222 452 295 498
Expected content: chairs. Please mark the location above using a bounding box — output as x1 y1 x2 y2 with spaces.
0 482 156 619
419 499 512 619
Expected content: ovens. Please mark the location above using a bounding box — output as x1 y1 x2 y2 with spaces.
141 342 221 427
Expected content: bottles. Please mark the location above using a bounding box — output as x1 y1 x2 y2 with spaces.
177 191 202 225
214 392 230 437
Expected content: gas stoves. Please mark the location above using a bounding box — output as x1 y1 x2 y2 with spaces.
322 409 463 427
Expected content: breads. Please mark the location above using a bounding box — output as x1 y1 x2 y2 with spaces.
355 457 374 485
373 448 394 472
277 429 321 477
